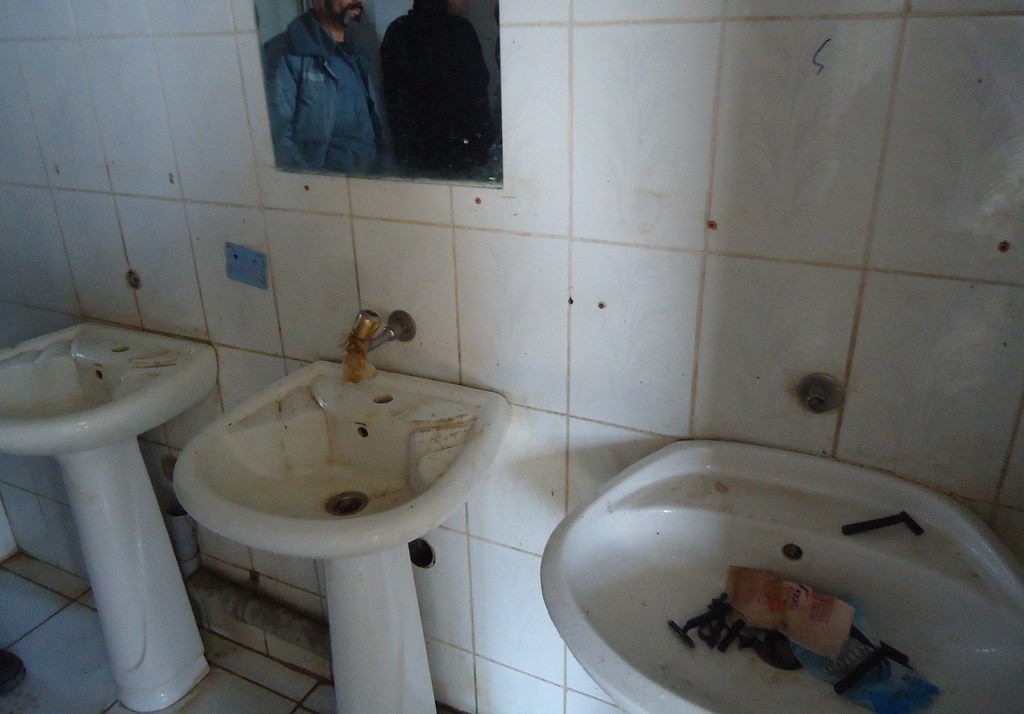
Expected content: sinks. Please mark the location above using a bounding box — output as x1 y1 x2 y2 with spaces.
171 358 515 559
0 321 218 457
540 438 1024 714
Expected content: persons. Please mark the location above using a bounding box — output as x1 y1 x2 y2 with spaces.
261 0 387 176
378 0 491 177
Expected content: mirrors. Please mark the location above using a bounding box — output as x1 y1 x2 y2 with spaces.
254 0 503 190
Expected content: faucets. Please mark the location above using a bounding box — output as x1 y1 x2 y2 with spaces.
342 309 418 354
